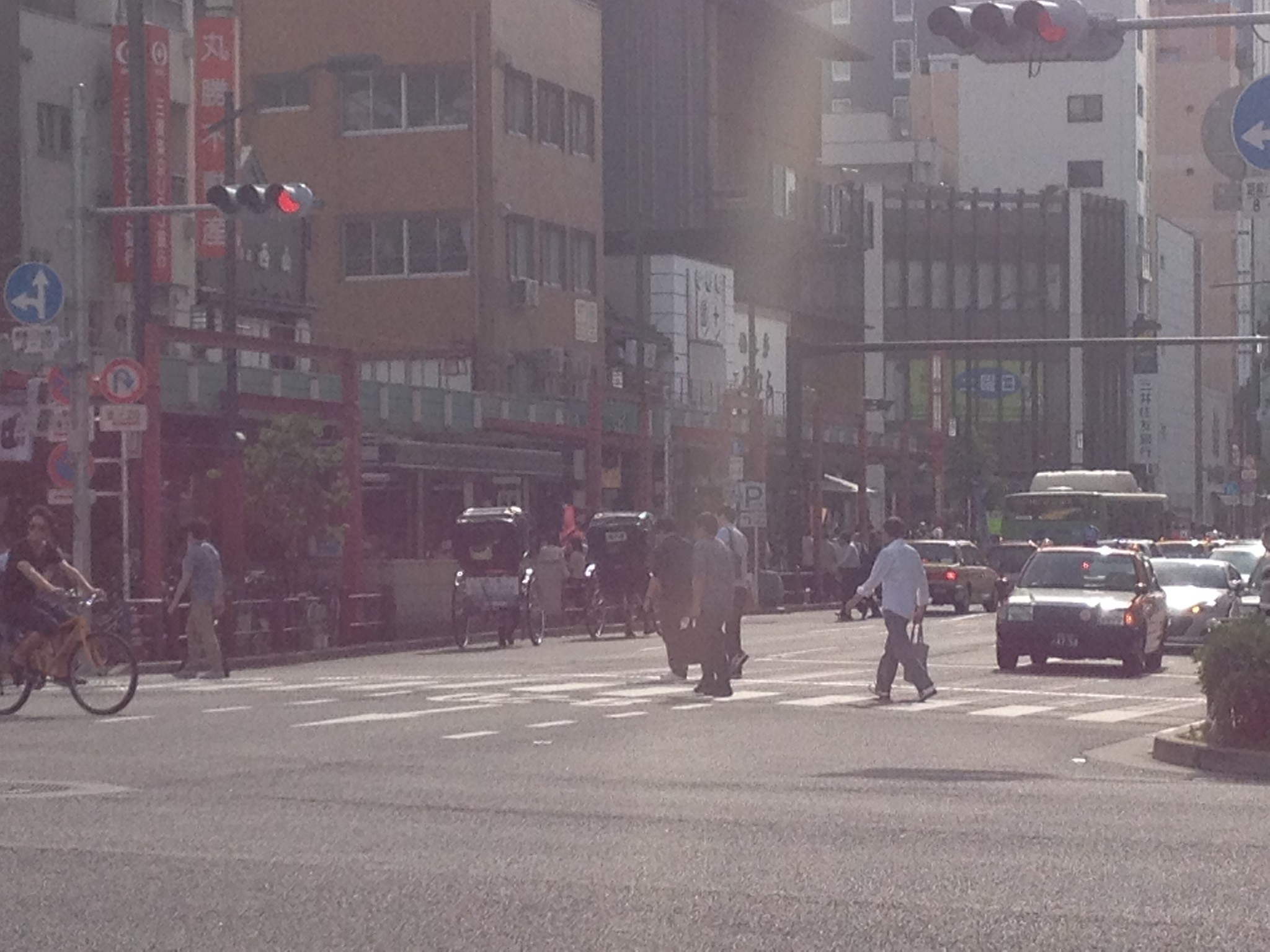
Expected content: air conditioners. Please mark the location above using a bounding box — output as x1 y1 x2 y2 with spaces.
515 278 539 306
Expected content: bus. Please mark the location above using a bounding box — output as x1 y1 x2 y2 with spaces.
1000 470 1170 548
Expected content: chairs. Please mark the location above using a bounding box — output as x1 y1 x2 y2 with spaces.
1104 572 1135 588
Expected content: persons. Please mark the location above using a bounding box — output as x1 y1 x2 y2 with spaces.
5 505 107 686
165 516 226 680
846 515 938 702
351 488 1270 699
687 511 735 698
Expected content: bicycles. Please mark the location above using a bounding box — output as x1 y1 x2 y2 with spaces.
0 588 140 718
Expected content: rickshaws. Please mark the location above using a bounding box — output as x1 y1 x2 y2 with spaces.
581 509 654 640
445 506 547 649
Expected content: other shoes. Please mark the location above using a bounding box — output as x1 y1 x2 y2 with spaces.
199 670 224 679
842 615 853 621
694 676 714 696
732 670 742 678
714 680 732 697
918 686 936 703
835 613 845 616
869 613 881 618
6 653 24 685
626 632 641 639
862 610 867 621
869 684 890 699
644 623 659 635
728 651 749 678
173 670 196 679
54 676 87 687
672 667 688 679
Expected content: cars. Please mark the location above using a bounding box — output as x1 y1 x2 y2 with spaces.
904 540 1000 615
991 538 1269 678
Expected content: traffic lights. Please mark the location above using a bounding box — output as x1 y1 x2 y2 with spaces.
205 183 314 220
919 0 1097 62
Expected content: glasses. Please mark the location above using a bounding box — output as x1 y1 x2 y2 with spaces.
30 524 45 532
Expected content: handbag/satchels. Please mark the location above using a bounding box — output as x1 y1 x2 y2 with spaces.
731 550 742 578
681 616 700 663
905 624 929 682
